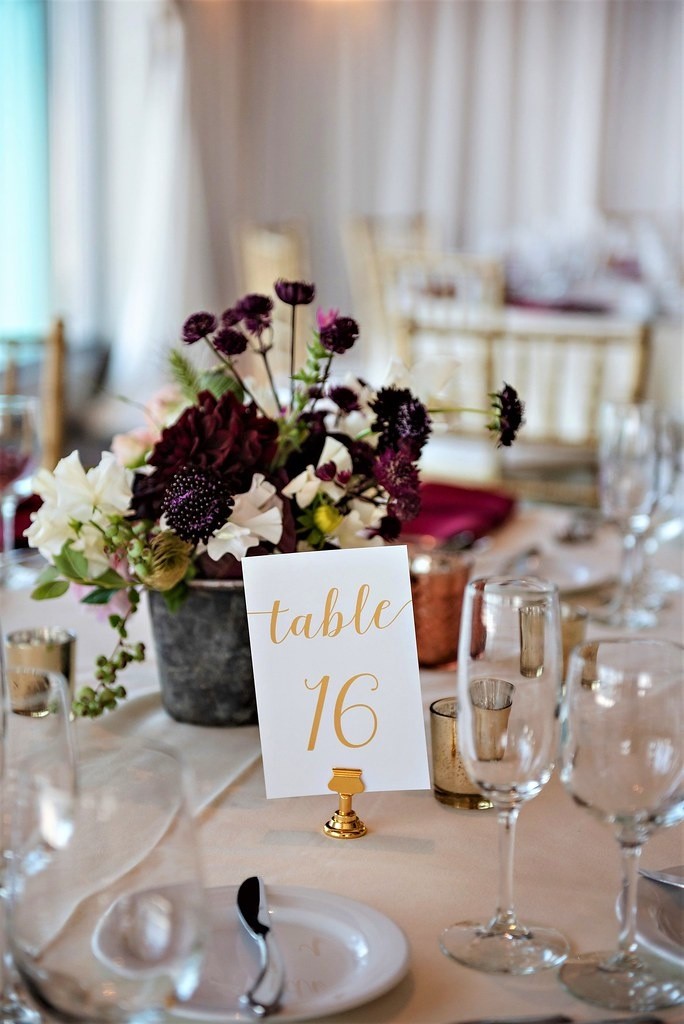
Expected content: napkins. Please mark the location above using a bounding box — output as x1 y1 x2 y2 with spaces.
403 483 518 547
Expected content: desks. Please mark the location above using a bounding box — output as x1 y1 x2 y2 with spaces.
0 513 684 1024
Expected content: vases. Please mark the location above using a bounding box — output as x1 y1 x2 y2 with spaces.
148 579 257 728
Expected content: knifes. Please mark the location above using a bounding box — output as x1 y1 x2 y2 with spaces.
233 876 285 1018
636 868 684 888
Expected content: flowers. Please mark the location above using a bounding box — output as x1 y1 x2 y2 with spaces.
24 277 525 716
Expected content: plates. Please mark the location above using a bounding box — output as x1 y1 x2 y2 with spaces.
468 502 621 590
108 882 411 1024
618 866 684 966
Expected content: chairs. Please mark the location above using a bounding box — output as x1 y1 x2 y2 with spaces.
0 319 65 504
224 217 655 508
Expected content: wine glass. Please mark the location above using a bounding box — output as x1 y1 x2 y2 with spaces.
557 638 684 1008
0 670 74 1024
590 402 673 629
0 395 43 589
443 578 570 976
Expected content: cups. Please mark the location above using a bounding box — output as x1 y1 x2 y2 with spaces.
10 746 204 1024
430 698 493 810
469 678 513 762
3 626 76 717
519 606 585 680
